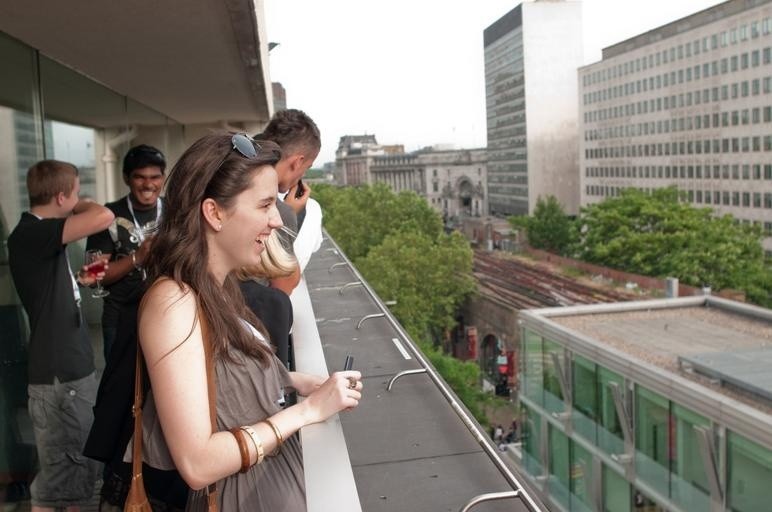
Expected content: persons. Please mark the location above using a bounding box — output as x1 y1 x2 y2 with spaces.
85 145 168 509
121 132 361 511
252 133 323 276
237 227 299 369
6 158 115 512
489 421 516 445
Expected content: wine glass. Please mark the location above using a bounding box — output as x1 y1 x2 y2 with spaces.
85 250 111 299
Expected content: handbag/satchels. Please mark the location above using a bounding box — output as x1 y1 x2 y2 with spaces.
119 476 219 512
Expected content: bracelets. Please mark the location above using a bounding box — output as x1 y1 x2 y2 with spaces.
240 425 264 465
132 253 139 269
257 419 282 457
229 426 250 474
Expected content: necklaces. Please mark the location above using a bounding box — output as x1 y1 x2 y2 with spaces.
127 193 163 243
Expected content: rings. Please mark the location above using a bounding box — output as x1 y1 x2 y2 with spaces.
347 378 356 389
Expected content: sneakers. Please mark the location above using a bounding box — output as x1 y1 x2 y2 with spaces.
0 482 19 511
14 481 32 507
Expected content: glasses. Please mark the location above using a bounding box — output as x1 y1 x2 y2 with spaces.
215 131 264 168
